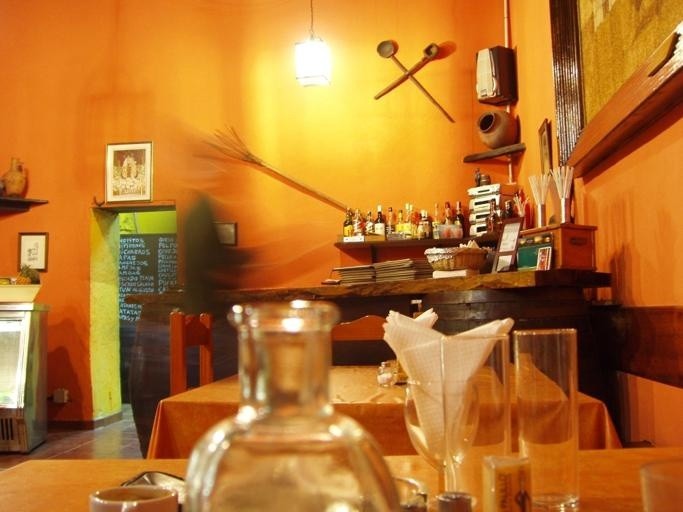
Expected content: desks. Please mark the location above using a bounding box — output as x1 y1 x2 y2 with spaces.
123 264 612 462
0 441 682 511
149 358 621 460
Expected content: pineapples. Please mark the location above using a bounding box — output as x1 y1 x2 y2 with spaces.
16 276 31 285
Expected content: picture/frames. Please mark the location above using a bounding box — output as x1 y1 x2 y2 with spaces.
546 1 683 178
535 118 553 175
487 217 525 273
16 230 49 274
214 221 238 247
103 138 157 202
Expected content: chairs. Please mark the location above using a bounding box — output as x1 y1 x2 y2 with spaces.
328 311 432 366
160 304 215 399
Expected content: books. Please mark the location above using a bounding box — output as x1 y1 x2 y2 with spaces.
320 257 416 284
433 269 480 278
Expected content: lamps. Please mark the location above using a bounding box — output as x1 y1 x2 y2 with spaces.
293 0 329 85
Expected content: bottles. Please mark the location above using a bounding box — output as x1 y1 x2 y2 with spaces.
343 201 464 241
486 198 499 231
182 299 405 512
501 200 514 220
377 361 395 387
3 157 26 198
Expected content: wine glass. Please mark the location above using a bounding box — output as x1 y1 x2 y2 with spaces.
402 376 479 491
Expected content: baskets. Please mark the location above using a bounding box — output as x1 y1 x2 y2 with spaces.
424 247 488 271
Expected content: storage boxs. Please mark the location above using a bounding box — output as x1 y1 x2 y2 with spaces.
0 283 42 304
518 221 598 272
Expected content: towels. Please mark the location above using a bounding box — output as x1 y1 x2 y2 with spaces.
385 305 513 446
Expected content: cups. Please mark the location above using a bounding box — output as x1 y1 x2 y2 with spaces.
89 485 182 512
640 456 682 512
513 327 579 512
483 455 534 509
426 493 475 512
392 475 424 510
443 334 509 510
